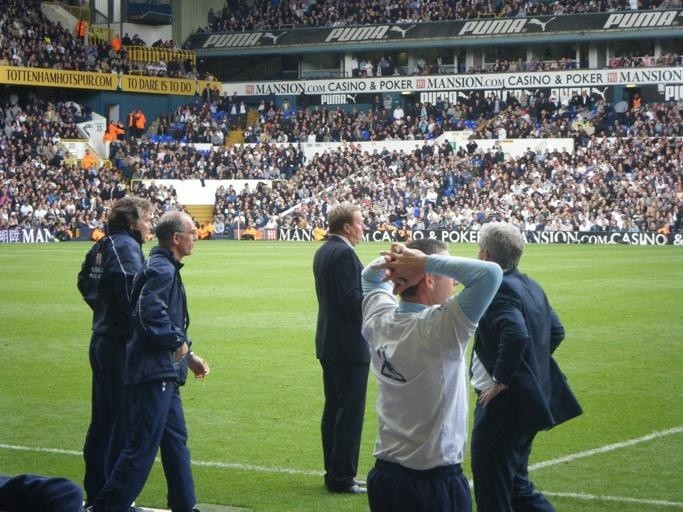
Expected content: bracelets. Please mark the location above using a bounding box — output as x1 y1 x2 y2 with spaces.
492 376 501 385
187 351 196 360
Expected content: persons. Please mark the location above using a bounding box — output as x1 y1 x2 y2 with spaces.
551 57 575 70
350 56 401 78
358 236 504 512
205 72 218 82
74 195 155 512
464 219 587 511
240 91 679 241
493 59 551 73
410 57 441 76
195 1 679 34
641 54 679 67
311 202 373 496
127 81 248 243
2 1 199 80
606 52 642 68
1 95 131 243
467 65 488 74
84 208 211 512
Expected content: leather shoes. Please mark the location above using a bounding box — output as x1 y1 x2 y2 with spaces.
325 477 368 494
